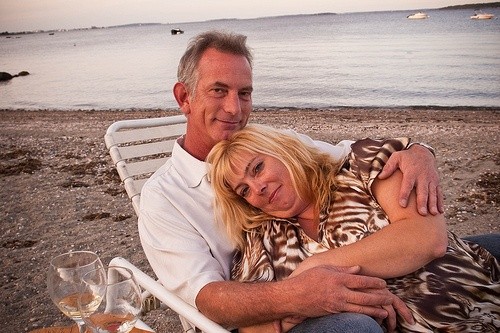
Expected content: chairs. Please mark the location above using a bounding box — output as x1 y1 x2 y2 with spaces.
101 114 232 333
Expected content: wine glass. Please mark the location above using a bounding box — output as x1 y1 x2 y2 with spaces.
47 251 107 333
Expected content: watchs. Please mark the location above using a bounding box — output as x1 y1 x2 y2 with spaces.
406 141 436 159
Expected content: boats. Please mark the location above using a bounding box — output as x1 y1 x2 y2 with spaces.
407 11 427 18
470 13 495 20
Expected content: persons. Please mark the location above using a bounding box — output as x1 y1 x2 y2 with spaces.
137 30 500 333
205 125 500 333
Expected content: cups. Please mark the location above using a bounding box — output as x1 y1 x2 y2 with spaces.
78 266 143 333
